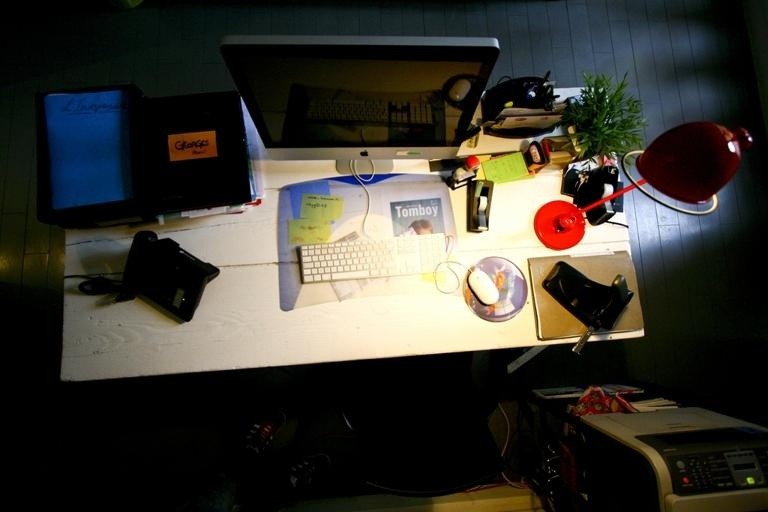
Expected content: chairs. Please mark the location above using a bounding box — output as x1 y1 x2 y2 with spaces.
317 400 521 499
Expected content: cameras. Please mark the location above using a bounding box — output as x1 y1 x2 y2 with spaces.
561 168 625 224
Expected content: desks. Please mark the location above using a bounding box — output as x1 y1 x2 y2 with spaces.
58 84 649 385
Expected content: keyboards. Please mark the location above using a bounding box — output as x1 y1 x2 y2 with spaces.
298 232 448 284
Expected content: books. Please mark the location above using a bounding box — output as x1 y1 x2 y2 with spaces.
533 380 680 413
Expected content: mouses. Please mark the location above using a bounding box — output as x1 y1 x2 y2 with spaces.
467 270 500 305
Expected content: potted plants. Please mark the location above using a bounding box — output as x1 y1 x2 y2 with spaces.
557 70 650 165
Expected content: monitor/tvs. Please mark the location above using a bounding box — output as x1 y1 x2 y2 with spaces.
217 33 500 177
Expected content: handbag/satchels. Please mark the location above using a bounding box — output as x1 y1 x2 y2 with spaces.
125 229 219 322
